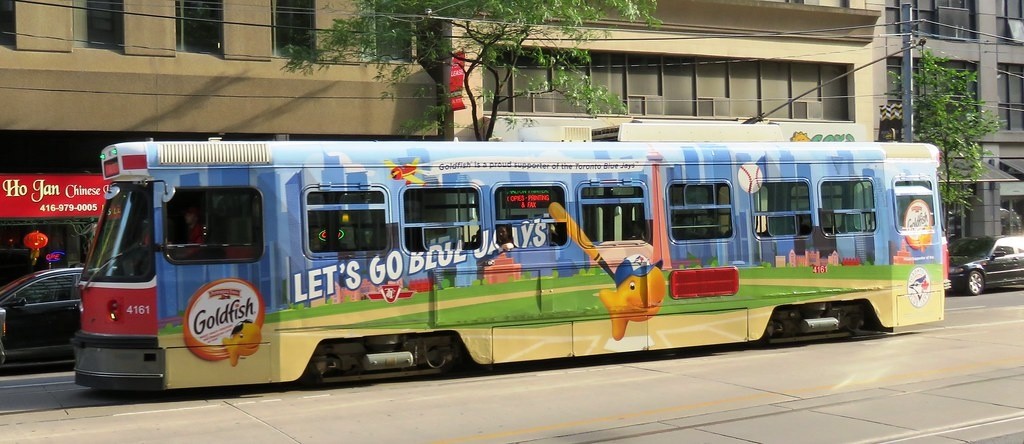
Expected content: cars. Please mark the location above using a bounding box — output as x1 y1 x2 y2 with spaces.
947 233 1024 296
0 267 119 375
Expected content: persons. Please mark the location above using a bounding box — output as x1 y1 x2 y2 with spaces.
171 203 205 258
466 225 516 251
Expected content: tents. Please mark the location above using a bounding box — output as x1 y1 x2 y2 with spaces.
7 236 17 248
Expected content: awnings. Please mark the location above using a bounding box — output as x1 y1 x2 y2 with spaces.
999 158 1024 173
936 159 1020 184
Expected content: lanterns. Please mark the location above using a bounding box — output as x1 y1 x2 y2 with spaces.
23 228 49 266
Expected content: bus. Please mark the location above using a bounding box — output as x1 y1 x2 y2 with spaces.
70 138 951 404
